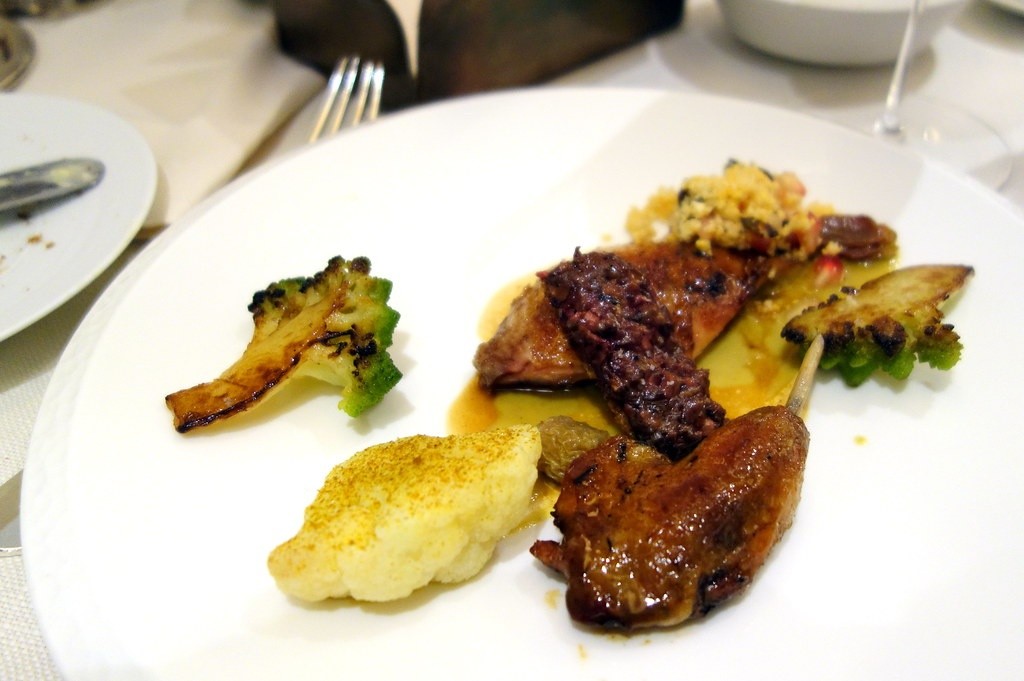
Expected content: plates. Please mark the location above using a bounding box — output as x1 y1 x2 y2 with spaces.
0 91 158 343
19 87 1024 681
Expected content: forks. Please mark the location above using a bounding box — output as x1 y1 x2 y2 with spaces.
308 40 387 146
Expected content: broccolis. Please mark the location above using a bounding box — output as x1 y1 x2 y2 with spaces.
163 255 403 432
780 263 972 386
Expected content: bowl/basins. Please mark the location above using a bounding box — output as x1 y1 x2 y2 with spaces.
720 0 953 66
